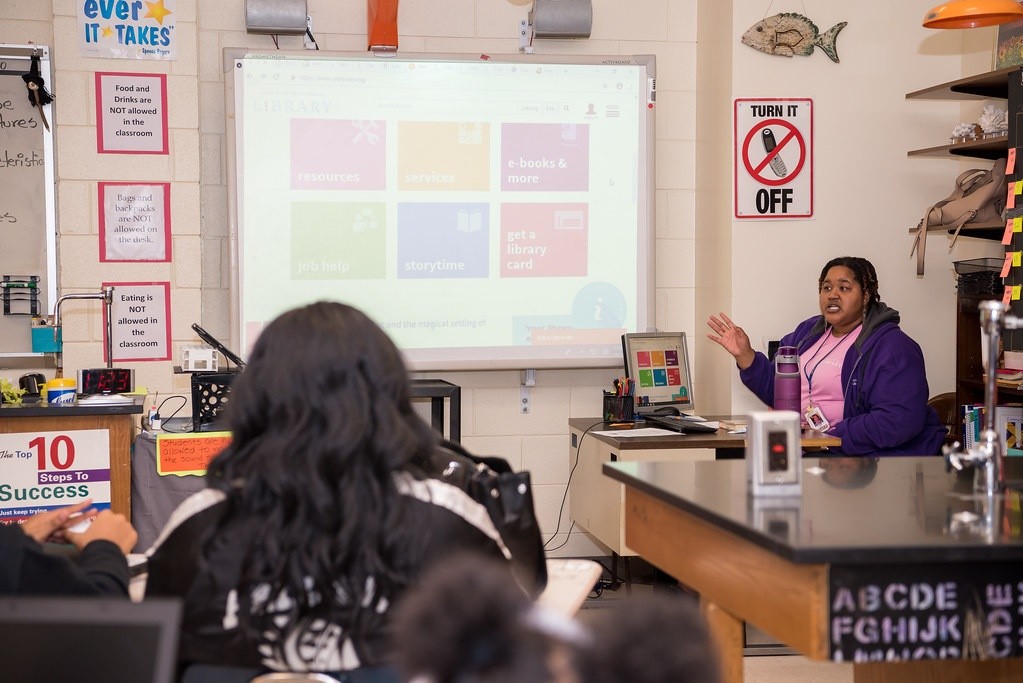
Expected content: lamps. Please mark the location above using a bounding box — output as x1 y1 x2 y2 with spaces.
922 0 1023 28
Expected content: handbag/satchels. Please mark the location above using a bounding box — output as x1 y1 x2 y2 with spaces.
429 437 547 604
908 158 1010 275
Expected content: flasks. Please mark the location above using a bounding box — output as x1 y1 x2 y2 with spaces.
774 346 801 413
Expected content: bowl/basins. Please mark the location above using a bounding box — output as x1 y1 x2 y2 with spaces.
19 374 46 395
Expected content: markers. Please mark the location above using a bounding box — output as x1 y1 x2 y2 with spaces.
1 282 36 288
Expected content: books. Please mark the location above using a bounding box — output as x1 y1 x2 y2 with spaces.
991 350 1023 385
961 403 1023 455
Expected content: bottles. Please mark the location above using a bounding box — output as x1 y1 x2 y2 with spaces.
148 405 162 430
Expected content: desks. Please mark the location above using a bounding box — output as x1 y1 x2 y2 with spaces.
0 395 144 544
603 452 1023 683
568 416 842 590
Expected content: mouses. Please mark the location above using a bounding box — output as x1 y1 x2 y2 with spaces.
654 407 680 415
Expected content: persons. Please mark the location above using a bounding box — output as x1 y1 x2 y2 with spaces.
375 547 722 683
143 302 534 683
706 256 929 452
0 496 139 600
812 414 822 426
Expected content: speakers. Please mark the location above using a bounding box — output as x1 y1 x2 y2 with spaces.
243 0 308 33
533 0 593 37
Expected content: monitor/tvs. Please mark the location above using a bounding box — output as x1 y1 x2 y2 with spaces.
622 332 694 416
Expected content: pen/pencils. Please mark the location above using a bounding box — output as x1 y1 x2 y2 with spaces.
609 423 634 426
614 376 635 420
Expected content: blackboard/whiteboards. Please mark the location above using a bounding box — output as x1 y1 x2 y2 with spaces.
0 45 59 359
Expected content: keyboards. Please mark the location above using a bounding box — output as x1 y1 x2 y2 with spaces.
643 414 718 434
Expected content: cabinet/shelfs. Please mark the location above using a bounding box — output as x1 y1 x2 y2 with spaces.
905 67 1023 238
